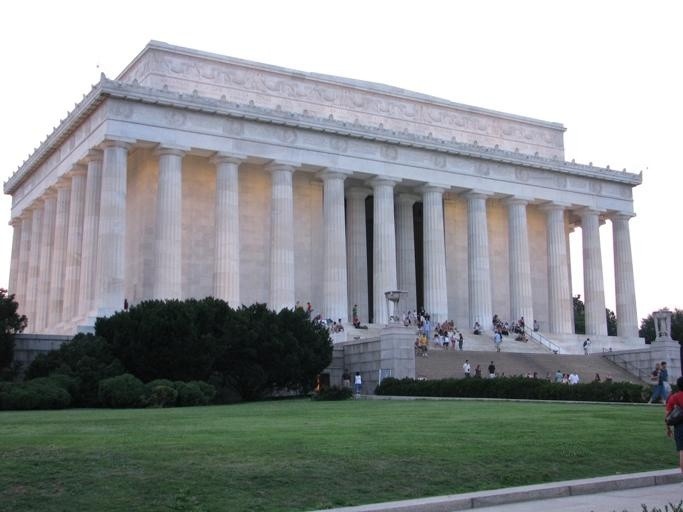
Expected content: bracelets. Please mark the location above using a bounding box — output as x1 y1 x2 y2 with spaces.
666 427 670 429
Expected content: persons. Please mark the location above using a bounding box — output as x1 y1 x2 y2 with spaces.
666 384 676 405
342 368 364 396
583 338 592 355
647 362 666 404
123 298 128 312
294 300 368 335
463 360 600 385
665 375 683 478
473 314 539 352
390 306 463 357
660 361 670 403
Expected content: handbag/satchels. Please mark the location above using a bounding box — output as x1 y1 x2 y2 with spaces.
664 407 682 426
